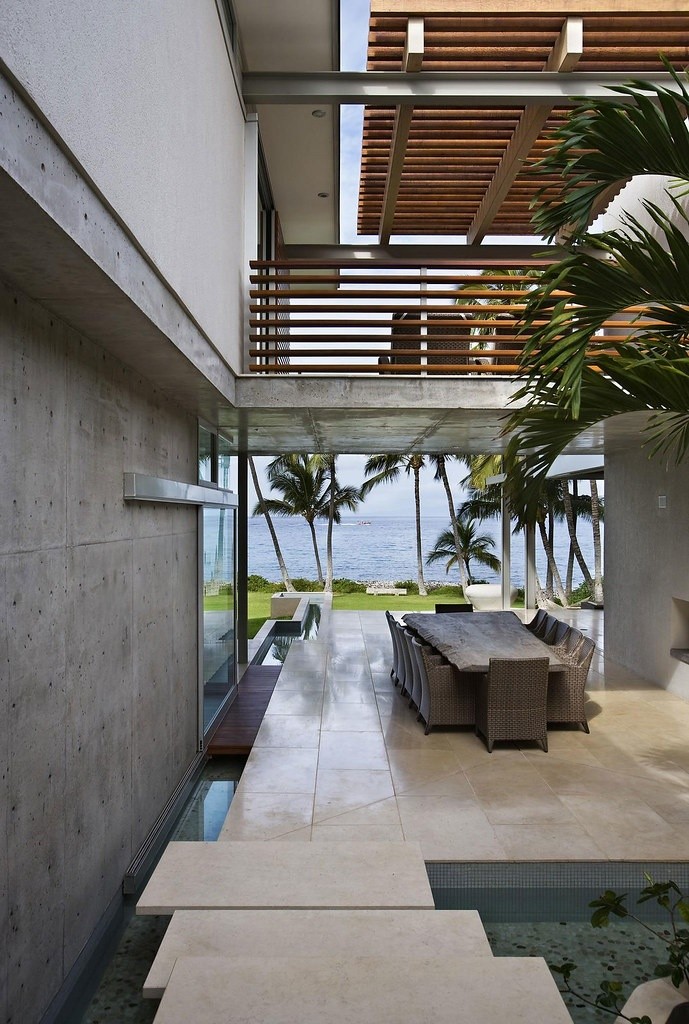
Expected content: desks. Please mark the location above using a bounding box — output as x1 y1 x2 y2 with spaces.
401 611 567 673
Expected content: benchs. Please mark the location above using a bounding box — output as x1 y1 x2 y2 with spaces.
366 588 406 596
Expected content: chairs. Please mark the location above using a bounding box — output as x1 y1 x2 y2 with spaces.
395 623 432 708
524 608 548 632
541 621 569 645
412 637 480 735
385 610 413 686
378 312 471 376
549 627 584 657
475 315 569 377
390 615 427 693
476 656 550 752
546 635 597 733
403 629 442 719
434 603 473 614
533 615 560 641
